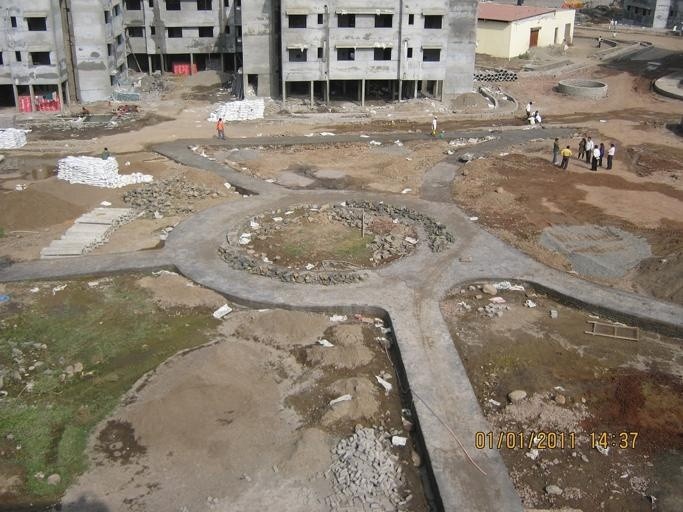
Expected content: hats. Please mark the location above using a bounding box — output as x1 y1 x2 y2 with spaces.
434 116 437 119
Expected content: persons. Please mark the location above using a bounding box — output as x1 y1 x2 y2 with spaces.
551 137 560 165
560 146 572 169
559 40 567 56
606 18 618 38
597 36 602 49
525 101 541 126
101 147 110 161
672 24 677 35
430 115 437 137
215 117 225 139
578 137 615 172
